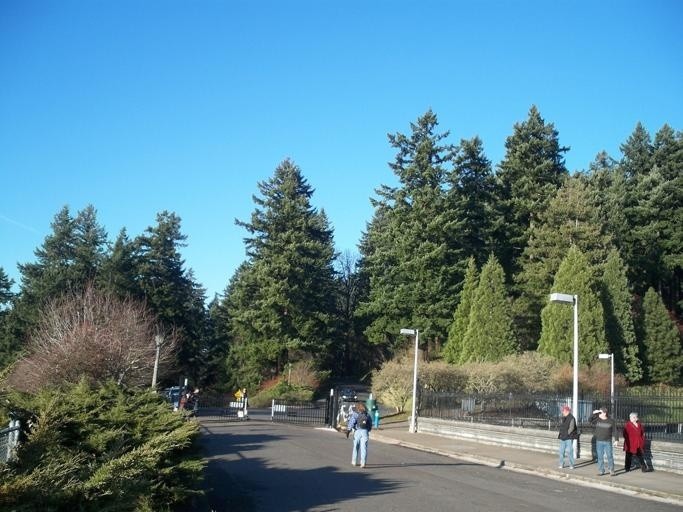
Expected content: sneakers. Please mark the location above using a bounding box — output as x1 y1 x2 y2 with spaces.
352 463 356 465
598 472 604 476
610 472 615 475
361 464 365 467
626 469 630 472
559 466 563 468
570 467 574 469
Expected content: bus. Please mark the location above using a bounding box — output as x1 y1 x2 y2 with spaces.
162 386 190 408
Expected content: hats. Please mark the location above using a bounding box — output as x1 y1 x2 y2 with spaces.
563 406 570 411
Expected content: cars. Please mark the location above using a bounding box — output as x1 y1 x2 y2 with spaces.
340 386 358 403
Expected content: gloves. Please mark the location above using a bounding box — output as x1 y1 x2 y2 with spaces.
347 431 350 438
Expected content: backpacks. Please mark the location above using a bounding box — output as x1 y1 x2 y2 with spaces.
357 412 369 429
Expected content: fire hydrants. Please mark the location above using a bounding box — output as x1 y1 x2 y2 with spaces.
373 410 381 429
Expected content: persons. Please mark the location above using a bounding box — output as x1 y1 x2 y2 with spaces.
556 405 579 470
345 402 372 469
622 411 651 472
242 388 249 408
586 404 618 476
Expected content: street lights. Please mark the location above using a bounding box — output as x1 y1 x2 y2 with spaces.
400 327 420 432
150 333 163 394
286 363 292 386
547 292 582 459
599 352 616 421
